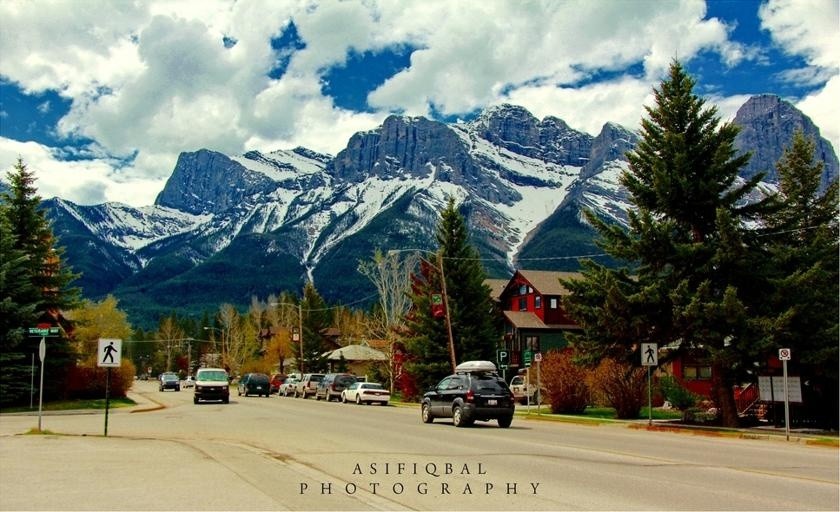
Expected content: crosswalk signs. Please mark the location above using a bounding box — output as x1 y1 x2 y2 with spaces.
97 338 122 366
642 343 658 367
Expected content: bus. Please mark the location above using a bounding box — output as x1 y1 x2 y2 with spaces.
654 346 758 401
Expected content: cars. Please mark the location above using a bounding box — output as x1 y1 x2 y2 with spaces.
421 360 551 426
159 368 390 407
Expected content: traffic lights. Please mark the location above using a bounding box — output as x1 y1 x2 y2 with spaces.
501 332 513 341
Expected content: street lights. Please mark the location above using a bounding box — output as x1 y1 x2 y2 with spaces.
271 302 304 381
140 326 225 368
389 249 458 373
